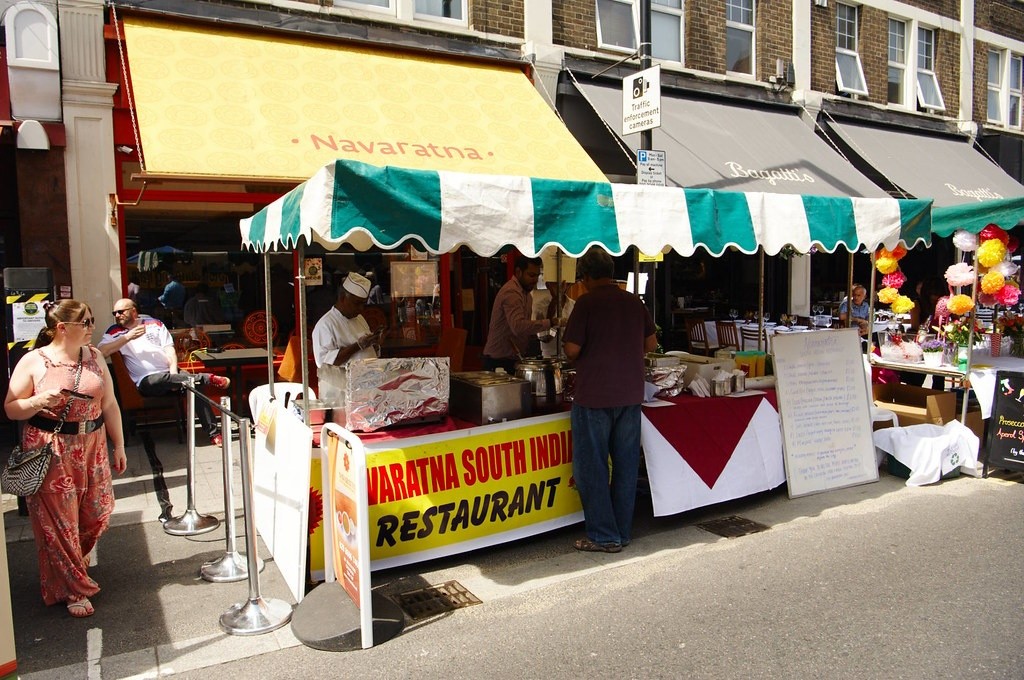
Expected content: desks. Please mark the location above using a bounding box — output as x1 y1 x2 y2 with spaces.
871 355 1024 426
193 345 276 426
689 319 833 359
799 321 912 343
368 332 433 357
639 389 780 521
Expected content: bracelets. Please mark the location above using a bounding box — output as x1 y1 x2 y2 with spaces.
111 447 125 451
29 397 40 412
356 340 364 351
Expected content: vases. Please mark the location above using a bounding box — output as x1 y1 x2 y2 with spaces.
923 352 941 367
958 345 971 364
1007 333 1024 359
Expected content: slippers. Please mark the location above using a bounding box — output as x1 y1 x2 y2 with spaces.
67 599 94 617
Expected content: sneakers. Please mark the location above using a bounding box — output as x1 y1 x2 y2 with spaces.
207 373 230 389
210 434 223 448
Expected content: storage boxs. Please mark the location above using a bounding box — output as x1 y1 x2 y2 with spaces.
871 384 957 425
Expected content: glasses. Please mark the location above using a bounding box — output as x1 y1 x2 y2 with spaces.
112 308 133 317
60 316 96 328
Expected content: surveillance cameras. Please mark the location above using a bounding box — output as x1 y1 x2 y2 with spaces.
121 146 133 153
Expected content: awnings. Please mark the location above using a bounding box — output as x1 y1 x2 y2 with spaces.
117 14 1023 206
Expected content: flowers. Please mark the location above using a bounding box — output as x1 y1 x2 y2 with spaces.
779 243 818 260
920 341 945 353
937 318 989 346
994 310 1024 333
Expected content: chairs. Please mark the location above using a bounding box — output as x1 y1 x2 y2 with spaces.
250 383 315 427
740 327 767 352
785 315 813 328
105 325 195 446
715 321 738 350
393 326 471 374
685 317 725 355
863 361 902 430
830 318 846 331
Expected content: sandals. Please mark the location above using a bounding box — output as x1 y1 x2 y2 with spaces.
622 539 630 546
572 538 622 553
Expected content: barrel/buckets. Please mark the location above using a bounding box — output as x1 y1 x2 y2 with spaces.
732 375 745 392
712 378 733 396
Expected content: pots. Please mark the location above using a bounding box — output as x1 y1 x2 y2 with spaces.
514 356 562 396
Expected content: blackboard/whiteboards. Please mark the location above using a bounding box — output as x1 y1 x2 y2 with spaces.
770 328 876 501
986 371 1024 471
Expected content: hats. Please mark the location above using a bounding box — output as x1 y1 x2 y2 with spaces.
343 271 371 298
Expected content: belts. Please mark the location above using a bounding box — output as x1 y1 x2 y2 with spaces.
28 413 105 435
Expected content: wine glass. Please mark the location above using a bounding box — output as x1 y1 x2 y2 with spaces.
813 305 818 316
819 306 824 316
729 308 738 321
780 314 786 325
754 311 759 323
764 313 770 326
812 316 819 331
825 317 832 329
791 315 797 329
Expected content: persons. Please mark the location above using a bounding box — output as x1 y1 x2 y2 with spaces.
483 254 570 375
837 285 877 351
97 299 230 447
362 268 383 304
560 247 657 554
18 300 63 518
184 281 228 325
158 270 197 315
4 298 128 617
312 270 382 399
128 273 160 316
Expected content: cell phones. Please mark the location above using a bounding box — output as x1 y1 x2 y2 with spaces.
373 325 387 337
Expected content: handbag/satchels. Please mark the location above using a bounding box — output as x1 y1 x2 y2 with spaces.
2 445 51 496
